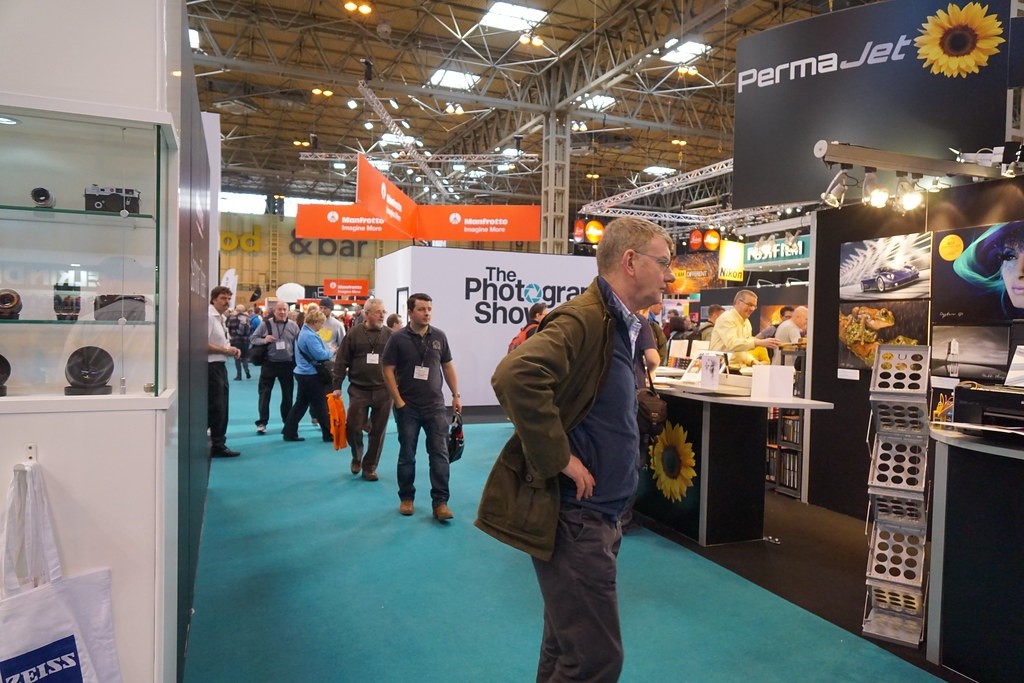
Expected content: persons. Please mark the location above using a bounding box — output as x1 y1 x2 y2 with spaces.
250 299 300 433
316 296 346 362
282 311 335 443
953 224 1024 320
754 305 794 364
332 299 396 480
708 289 781 376
471 215 675 683
639 298 725 366
521 302 548 340
387 313 402 331
771 305 807 365
208 287 242 457
379 292 463 520
224 297 365 382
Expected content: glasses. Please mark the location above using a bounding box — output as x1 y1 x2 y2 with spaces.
740 300 758 309
637 251 671 269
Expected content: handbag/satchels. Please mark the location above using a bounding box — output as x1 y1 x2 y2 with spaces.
637 387 667 434
316 359 332 384
449 411 464 463
250 319 272 366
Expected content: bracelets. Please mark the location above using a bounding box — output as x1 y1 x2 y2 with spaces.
452 392 460 398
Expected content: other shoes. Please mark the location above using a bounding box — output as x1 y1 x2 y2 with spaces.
323 435 334 441
311 418 317 426
284 435 305 441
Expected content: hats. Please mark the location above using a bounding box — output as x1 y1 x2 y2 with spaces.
319 298 334 310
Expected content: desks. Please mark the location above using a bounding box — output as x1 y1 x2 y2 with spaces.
627 364 835 547
915 423 1023 683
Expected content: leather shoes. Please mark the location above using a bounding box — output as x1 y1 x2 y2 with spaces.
362 469 378 480
433 505 453 520
212 446 240 457
351 460 361 473
400 500 414 515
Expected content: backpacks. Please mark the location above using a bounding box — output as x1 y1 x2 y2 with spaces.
686 323 714 356
508 323 539 355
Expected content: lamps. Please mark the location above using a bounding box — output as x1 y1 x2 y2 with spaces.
818 151 933 213
189 2 726 202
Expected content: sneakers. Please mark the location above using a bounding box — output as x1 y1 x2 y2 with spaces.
257 425 267 433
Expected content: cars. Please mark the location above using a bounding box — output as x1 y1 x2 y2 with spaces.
860 264 921 293
